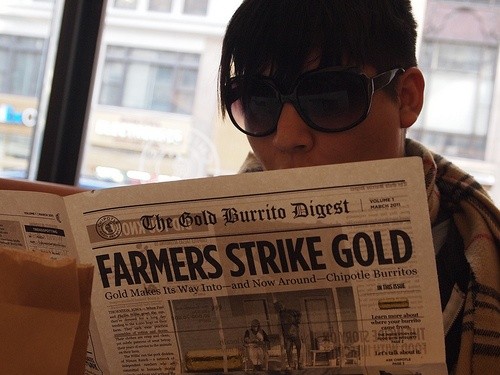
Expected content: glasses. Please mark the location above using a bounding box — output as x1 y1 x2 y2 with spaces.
223 66 405 138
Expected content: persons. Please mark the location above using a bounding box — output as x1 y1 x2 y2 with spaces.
213 0 500 375
244 320 270 371
276 301 303 371
